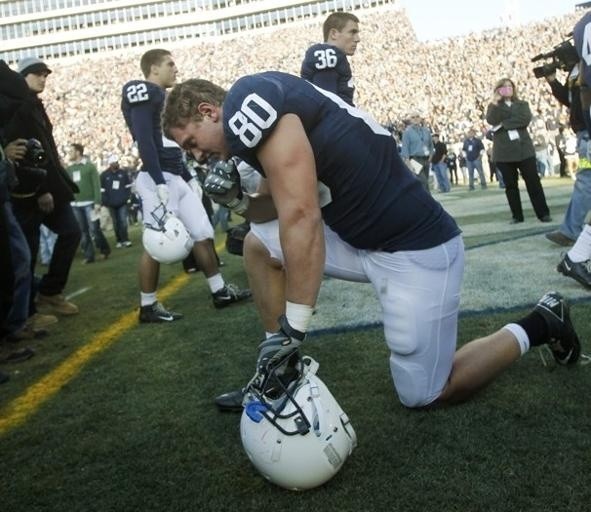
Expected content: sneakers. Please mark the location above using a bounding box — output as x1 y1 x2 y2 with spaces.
139 300 182 323
1 345 35 363
211 282 251 309
540 215 552 222
28 312 57 328
214 386 242 411
557 253 591 289
545 229 573 246
8 324 48 342
509 218 522 224
536 291 581 366
36 294 79 316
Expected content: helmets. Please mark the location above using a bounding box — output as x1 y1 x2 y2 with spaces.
142 205 193 266
239 356 357 493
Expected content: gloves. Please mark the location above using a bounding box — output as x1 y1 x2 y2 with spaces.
155 184 171 205
256 315 307 379
202 161 243 209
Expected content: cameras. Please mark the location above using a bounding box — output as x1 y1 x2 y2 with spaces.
24 138 45 169
531 37 580 79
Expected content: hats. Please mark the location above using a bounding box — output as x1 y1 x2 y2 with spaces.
17 57 52 77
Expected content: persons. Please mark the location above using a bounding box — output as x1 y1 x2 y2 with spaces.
16 56 82 328
554 127 579 180
447 147 458 186
101 154 133 248
301 12 361 108
63 143 112 264
486 142 498 182
544 9 591 247
430 134 450 192
0 59 49 362
0 138 28 384
461 129 487 191
120 49 251 323
159 71 582 412
457 147 467 185
485 77 553 224
401 111 432 193
557 218 591 289
